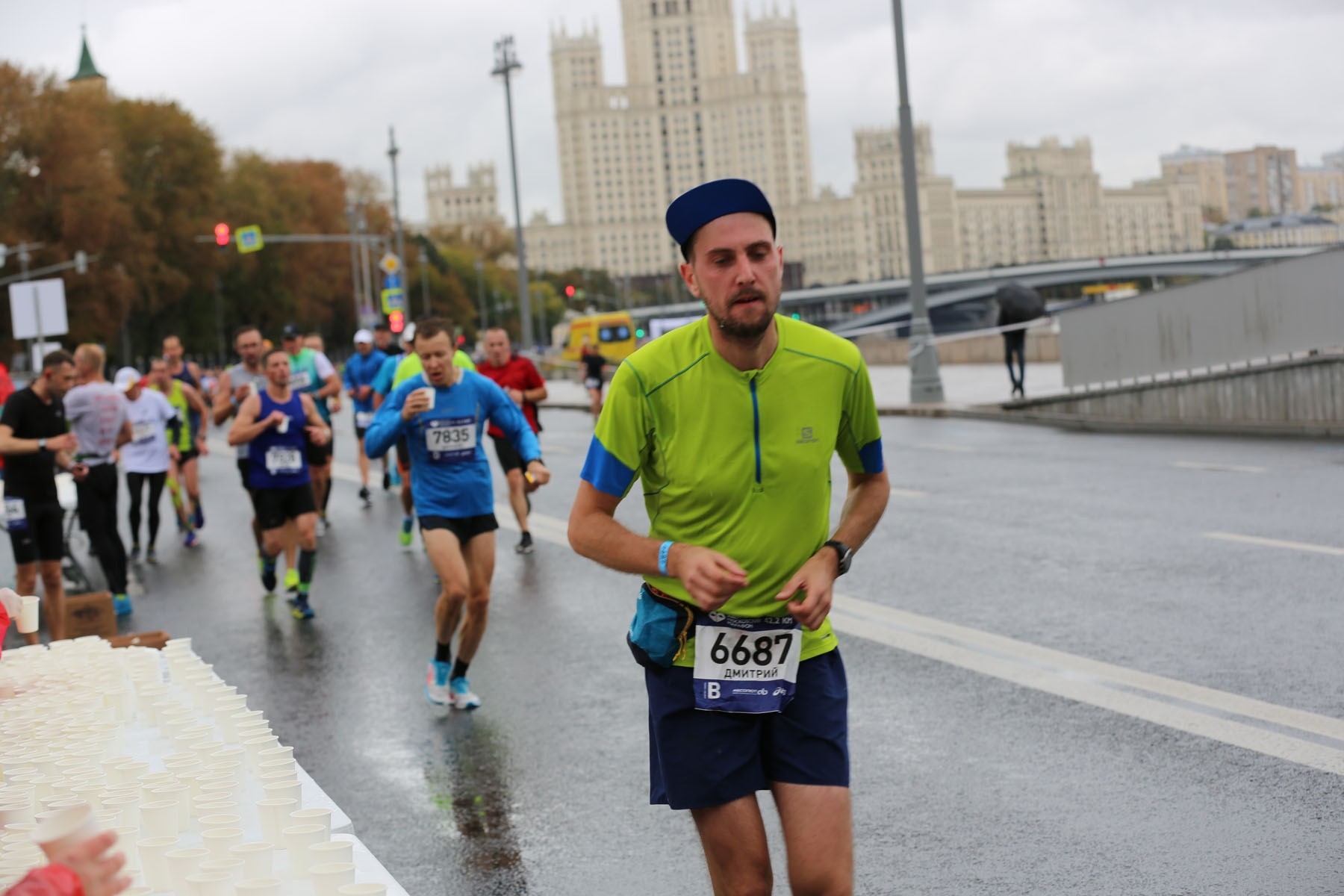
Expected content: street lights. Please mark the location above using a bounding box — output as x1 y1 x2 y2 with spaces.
490 34 539 349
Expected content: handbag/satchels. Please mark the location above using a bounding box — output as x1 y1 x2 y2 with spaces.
626 582 693 671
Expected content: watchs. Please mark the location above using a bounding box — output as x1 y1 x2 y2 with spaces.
37 436 48 454
823 539 855 579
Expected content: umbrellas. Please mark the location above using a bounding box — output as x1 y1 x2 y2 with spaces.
996 284 1044 323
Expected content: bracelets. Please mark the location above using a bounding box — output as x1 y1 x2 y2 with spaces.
313 390 321 404
522 390 526 402
196 433 207 441
658 539 673 575
65 458 77 471
228 394 240 410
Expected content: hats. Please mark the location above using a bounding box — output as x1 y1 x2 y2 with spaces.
282 322 298 339
353 328 373 344
113 366 142 393
665 178 777 247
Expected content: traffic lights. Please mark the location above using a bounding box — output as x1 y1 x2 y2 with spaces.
387 312 406 339
212 222 231 253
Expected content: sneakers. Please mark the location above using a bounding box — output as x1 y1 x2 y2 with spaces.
423 657 452 705
448 677 481 710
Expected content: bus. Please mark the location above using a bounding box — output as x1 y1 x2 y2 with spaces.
559 310 636 364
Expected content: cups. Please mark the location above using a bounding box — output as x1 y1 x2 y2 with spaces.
235 879 283 896
277 415 290 432
311 842 354 862
289 808 332 841
264 781 302 810
258 758 296 775
244 736 279 778
254 798 298 850
0 635 248 896
229 842 275 881
232 710 263 722
260 770 298 785
258 746 294 762
16 596 40 633
422 388 435 409
243 720 269 731
281 824 327 879
340 883 388 896
310 862 356 896
240 728 272 741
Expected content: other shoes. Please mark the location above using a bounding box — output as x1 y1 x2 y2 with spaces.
400 515 414 545
401 484 414 516
383 472 390 489
185 531 196 547
130 546 140 561
291 593 314 620
179 521 188 532
146 549 158 564
316 516 330 536
515 530 534 555
258 550 277 592
1011 382 1024 395
113 591 132 617
283 568 299 591
193 506 204 528
359 487 370 501
524 495 531 516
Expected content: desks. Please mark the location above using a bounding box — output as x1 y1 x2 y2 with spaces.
119 649 410 896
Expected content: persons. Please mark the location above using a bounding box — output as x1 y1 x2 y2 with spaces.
1 830 133 896
0 335 217 649
211 322 343 623
567 177 891 896
578 335 608 422
994 305 1027 396
345 315 550 711
